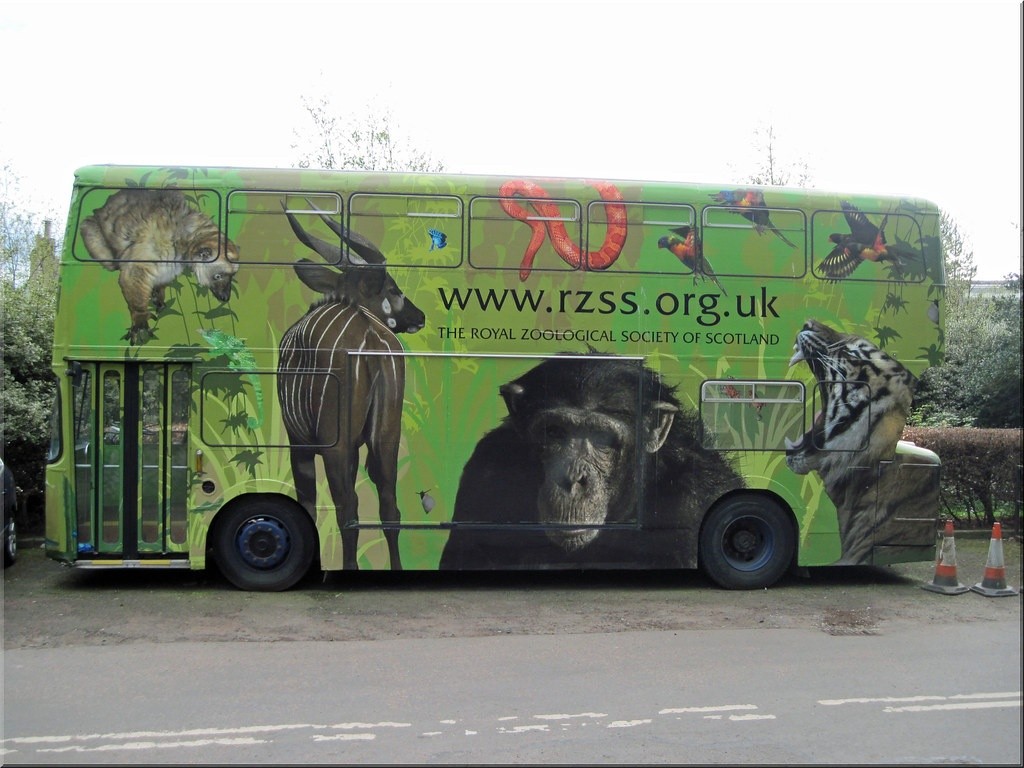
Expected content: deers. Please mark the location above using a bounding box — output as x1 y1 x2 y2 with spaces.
274 196 428 572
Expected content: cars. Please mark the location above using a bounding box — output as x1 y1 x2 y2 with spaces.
0 457 18 565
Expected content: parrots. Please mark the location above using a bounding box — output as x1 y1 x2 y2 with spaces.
657 224 729 297
708 188 799 250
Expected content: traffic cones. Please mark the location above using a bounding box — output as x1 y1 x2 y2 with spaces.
923 518 970 596
969 522 1018 597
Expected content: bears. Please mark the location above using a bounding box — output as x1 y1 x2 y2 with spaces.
77 184 243 346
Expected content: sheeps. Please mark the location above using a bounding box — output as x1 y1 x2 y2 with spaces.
413 489 435 516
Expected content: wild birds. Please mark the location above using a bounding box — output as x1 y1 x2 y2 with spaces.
854 202 899 266
813 198 887 284
925 297 940 327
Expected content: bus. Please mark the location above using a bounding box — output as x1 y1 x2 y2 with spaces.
35 160 950 588
